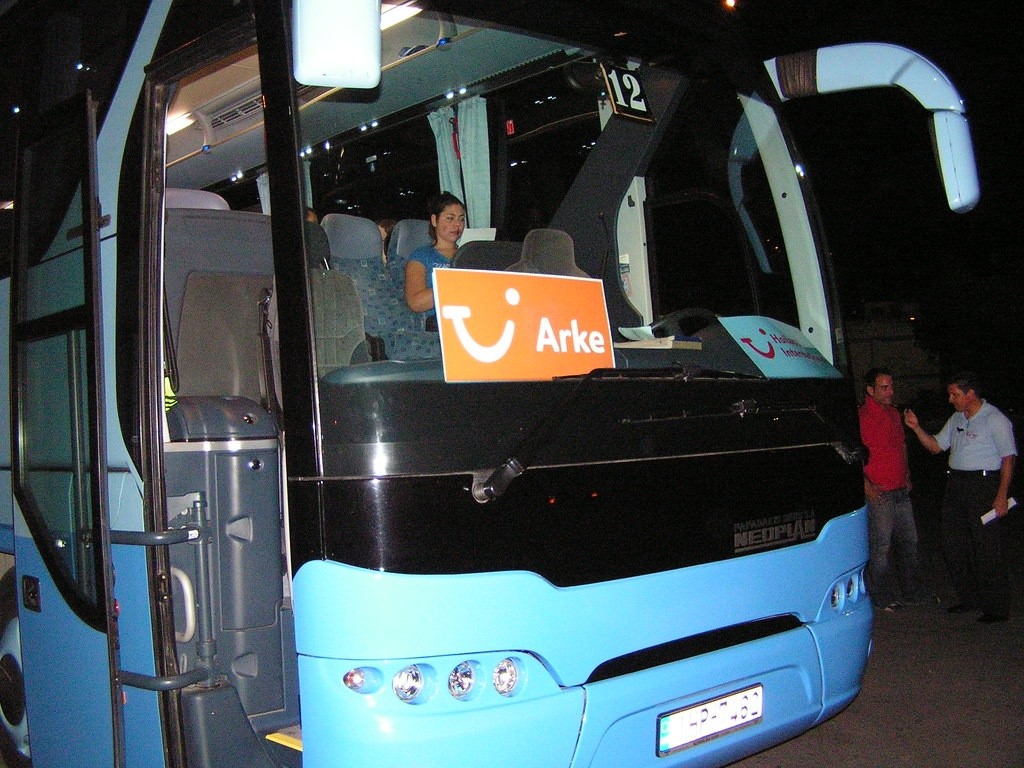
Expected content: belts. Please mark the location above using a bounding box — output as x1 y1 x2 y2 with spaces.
945 467 999 480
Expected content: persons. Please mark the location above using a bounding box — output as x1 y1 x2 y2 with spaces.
266 190 467 412
903 367 1019 626
854 369 941 612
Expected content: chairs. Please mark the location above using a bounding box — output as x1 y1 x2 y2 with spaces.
162 187 524 409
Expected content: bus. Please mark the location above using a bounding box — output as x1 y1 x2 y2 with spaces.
0 0 984 768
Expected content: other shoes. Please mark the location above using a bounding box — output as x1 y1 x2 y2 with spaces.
976 614 1009 623
874 601 905 612
948 602 979 613
